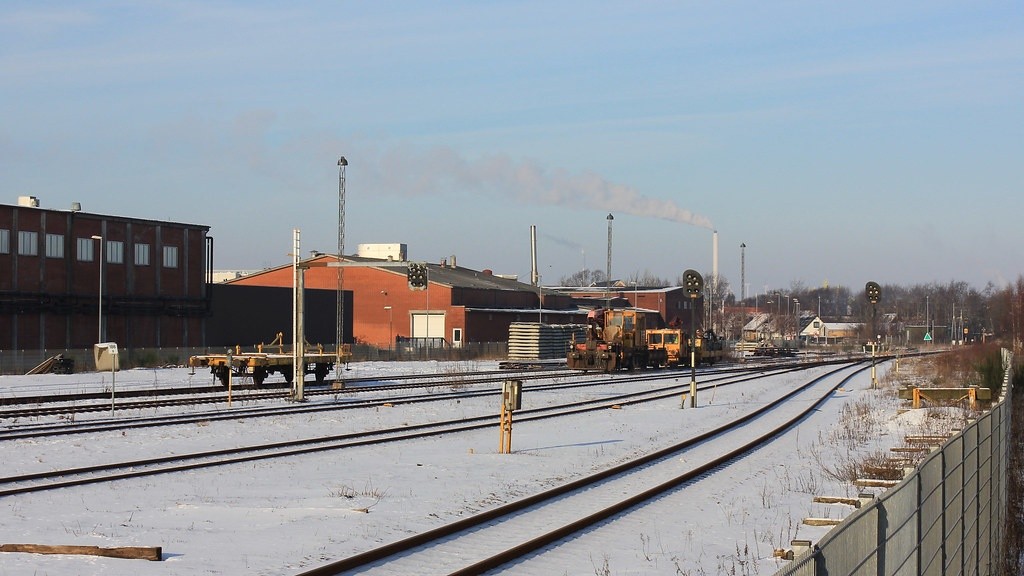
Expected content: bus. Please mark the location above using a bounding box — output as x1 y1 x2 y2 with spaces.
567 305 723 375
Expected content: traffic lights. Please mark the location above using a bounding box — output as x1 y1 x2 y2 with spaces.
683 269 703 300
865 281 880 304
406 263 427 290
